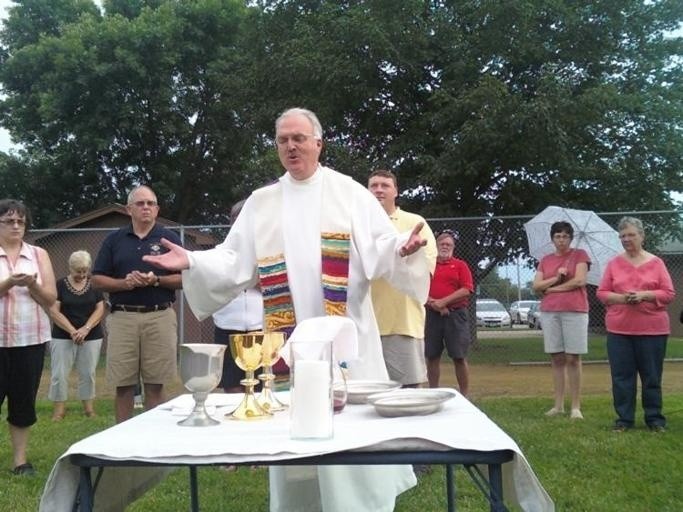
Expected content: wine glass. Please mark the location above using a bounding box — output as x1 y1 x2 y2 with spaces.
223 333 273 420
174 343 225 427
250 328 288 414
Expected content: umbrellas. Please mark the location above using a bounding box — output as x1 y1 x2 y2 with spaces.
521 206 632 289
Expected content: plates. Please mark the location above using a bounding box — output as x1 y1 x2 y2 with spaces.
331 379 400 403
364 387 455 416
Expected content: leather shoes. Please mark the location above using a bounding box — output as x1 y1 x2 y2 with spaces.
545 408 564 416
570 409 583 419
611 424 628 433
14 463 35 479
649 425 666 434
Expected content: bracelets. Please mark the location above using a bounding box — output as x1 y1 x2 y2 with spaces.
82 325 91 332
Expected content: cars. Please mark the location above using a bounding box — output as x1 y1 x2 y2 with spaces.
496 300 512 328
475 298 509 330
509 300 542 324
527 302 542 330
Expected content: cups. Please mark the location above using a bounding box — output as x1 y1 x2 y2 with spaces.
289 339 338 441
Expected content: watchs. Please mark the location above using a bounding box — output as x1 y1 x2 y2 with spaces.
151 273 160 289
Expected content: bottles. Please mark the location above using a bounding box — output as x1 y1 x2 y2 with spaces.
323 343 347 414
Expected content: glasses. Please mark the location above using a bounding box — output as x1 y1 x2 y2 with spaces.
127 199 156 207
278 132 313 144
0 219 24 225
436 228 460 247
553 235 571 239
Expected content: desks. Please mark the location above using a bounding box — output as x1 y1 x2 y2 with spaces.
38 387 555 512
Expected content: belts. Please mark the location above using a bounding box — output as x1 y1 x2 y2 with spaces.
112 304 166 313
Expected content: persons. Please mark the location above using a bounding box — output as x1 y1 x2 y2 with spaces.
422 231 477 402
101 289 145 410
531 220 593 422
208 197 264 474
0 196 62 478
362 168 439 477
591 215 677 433
47 248 106 424
140 106 428 512
84 185 185 426
